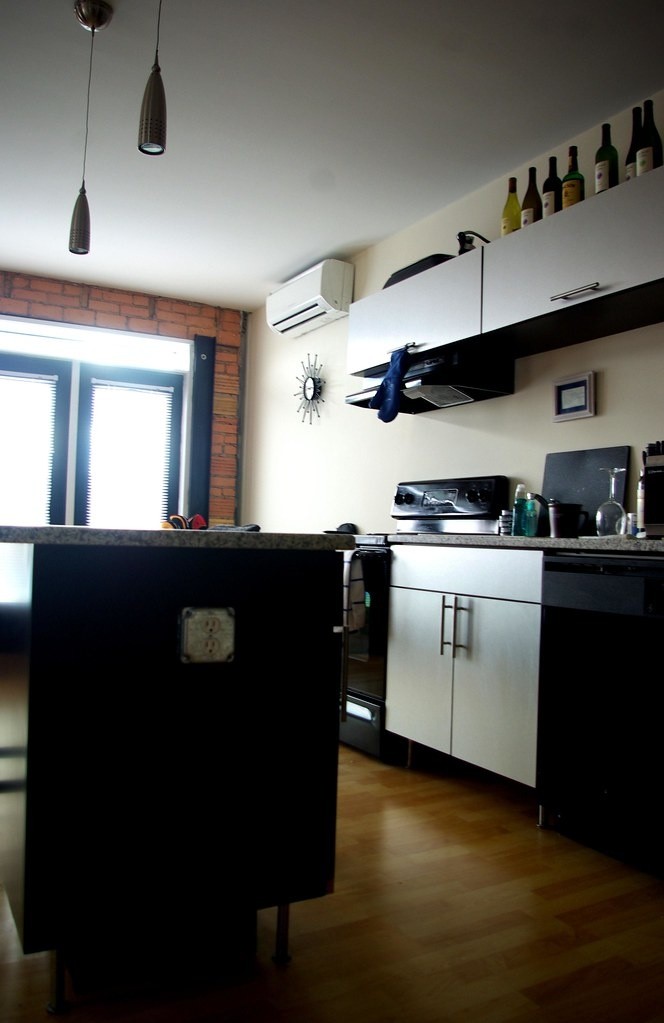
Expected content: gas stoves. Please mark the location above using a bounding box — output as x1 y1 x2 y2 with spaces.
336 472 508 549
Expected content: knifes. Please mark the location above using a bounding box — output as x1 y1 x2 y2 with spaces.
642 440 664 466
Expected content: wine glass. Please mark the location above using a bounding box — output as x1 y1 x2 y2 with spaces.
594 466 627 537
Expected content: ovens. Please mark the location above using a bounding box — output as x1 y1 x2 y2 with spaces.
329 546 392 737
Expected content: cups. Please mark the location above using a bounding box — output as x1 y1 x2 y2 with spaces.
547 502 589 538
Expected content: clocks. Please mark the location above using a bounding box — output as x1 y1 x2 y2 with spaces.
293 352 326 425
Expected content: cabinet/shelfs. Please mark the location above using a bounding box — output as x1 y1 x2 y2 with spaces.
384 543 547 789
482 165 664 357
535 551 664 877
0 523 357 1015
348 245 522 378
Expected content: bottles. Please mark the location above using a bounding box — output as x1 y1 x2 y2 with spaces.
542 156 563 220
510 483 538 538
625 107 644 182
501 177 522 238
521 167 543 228
636 100 663 180
594 123 619 196
562 146 585 210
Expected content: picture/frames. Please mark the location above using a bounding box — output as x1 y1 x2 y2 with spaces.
551 370 598 422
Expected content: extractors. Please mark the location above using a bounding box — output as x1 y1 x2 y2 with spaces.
344 340 518 414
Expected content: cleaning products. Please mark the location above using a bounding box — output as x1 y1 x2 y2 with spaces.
498 483 539 536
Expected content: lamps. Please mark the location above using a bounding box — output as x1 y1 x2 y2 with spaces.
69 0 114 255
137 0 168 155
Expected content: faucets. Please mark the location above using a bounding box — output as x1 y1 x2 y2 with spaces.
526 492 561 537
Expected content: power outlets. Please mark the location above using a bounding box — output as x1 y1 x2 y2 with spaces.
180 607 235 664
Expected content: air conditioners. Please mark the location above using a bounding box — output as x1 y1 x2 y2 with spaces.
266 259 354 338
